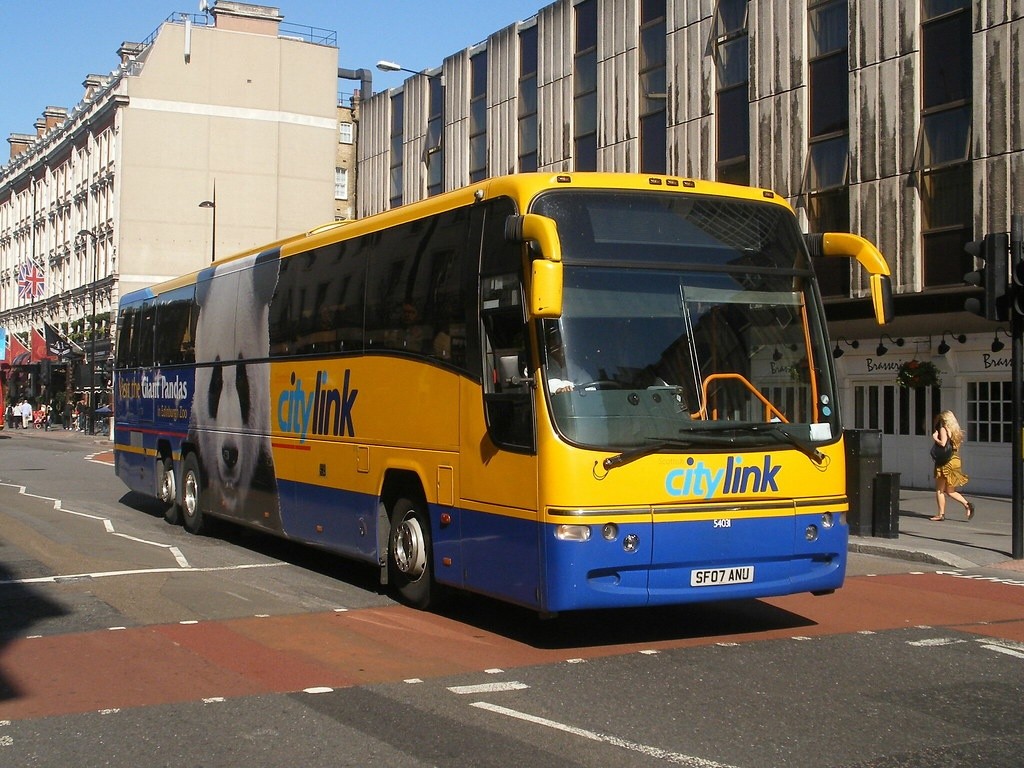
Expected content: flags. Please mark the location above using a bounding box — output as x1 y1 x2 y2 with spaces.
0 347 10 364
31 327 56 360
18 258 45 299
9 334 38 365
44 321 73 356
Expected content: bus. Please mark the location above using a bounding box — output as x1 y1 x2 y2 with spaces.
111 171 895 614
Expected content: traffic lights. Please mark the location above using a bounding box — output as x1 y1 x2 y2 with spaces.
963 232 1010 326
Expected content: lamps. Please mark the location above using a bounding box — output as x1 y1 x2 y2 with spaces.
833 336 859 358
772 339 798 361
938 330 967 354
991 327 1012 353
876 333 905 357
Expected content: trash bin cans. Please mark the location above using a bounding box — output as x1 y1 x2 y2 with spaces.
103 419 110 435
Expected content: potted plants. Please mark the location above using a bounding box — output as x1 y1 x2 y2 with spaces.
5 312 110 344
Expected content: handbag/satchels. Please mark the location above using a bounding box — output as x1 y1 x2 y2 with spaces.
930 426 953 464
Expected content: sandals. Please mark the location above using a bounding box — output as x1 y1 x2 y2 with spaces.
966 502 975 520
929 514 945 521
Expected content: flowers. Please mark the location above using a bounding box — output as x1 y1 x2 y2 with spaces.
894 358 948 394
785 355 810 386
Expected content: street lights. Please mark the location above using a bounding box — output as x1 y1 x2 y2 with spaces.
375 59 447 192
199 200 217 261
76 229 98 435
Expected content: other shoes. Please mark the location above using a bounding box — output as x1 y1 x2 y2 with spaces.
69 428 72 431
80 430 84 432
66 428 68 430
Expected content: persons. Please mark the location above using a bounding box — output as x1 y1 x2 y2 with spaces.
63 401 84 433
930 410 974 521
5 401 32 428
523 325 596 393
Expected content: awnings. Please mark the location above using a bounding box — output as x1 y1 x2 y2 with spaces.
72 363 112 393
